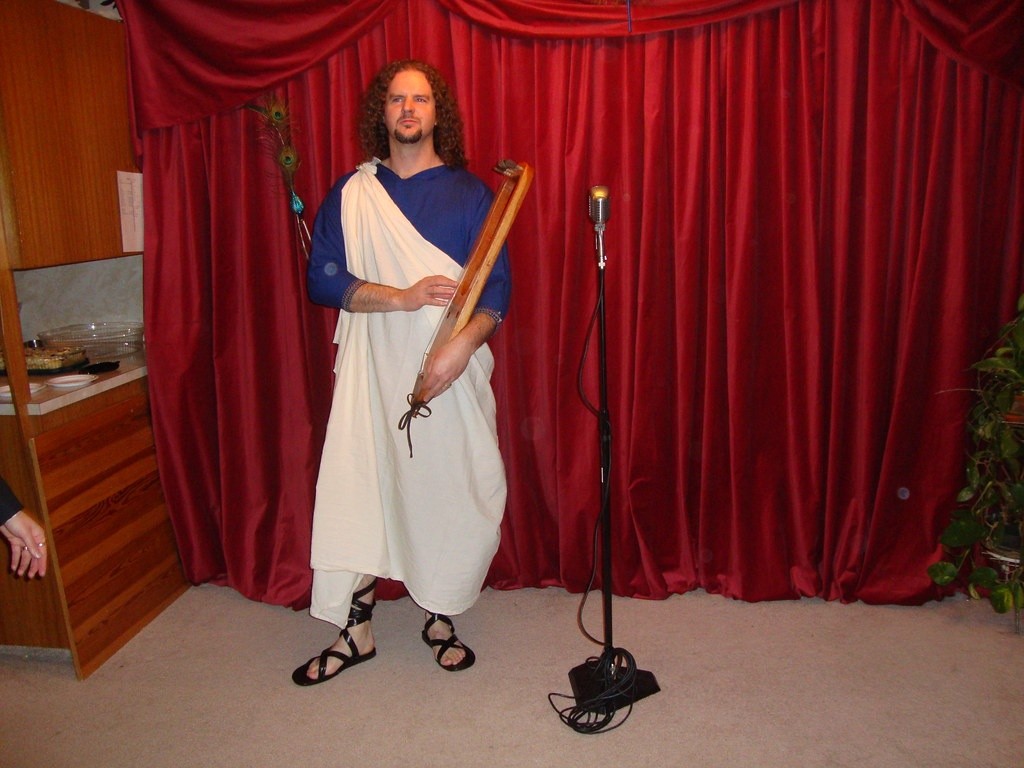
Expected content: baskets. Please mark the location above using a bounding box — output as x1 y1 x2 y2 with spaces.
0 347 86 370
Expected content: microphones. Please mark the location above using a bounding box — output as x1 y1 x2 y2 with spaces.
589 186 610 268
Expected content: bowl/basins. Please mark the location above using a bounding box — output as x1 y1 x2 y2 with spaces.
37 321 144 359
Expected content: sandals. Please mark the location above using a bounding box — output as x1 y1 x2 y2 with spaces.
292 578 378 686
422 612 475 671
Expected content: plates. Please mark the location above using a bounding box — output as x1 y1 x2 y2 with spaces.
0 382 41 397
44 374 99 388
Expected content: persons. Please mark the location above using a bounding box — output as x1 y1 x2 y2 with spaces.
0 476 47 578
292 59 512 687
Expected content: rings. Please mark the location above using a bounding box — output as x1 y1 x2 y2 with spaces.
23 547 28 550
38 543 45 546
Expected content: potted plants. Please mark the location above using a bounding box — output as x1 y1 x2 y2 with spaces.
924 295 1023 636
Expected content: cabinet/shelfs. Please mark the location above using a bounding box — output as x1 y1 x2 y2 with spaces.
0 0 144 271
